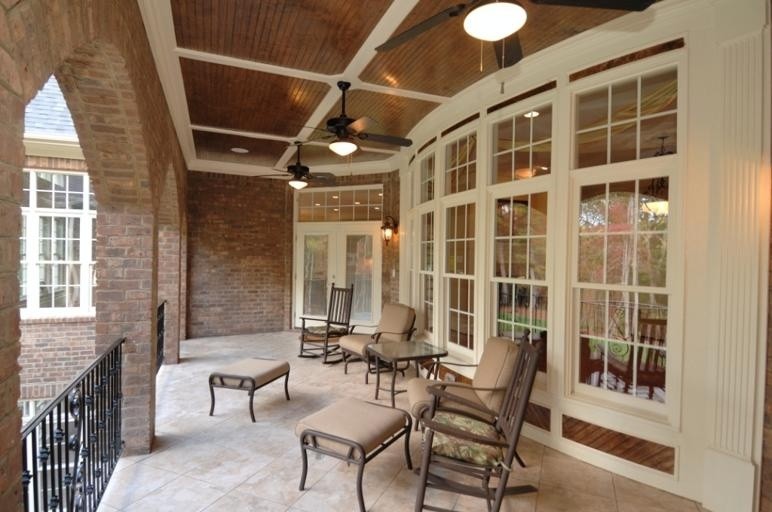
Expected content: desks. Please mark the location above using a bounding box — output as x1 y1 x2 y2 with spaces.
365 340 448 408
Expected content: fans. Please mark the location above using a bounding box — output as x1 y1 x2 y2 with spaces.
260 141 334 177
374 0 654 69
294 81 412 146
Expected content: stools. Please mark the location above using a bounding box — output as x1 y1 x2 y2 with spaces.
209 357 290 422
294 396 413 512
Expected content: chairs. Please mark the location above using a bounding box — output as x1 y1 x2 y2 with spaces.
339 303 417 384
581 332 603 385
298 282 353 364
414 328 545 512
407 336 520 431
598 308 667 399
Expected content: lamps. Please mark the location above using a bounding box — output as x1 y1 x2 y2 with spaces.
381 216 399 247
288 177 308 190
329 139 358 156
461 0 527 95
641 136 675 218
